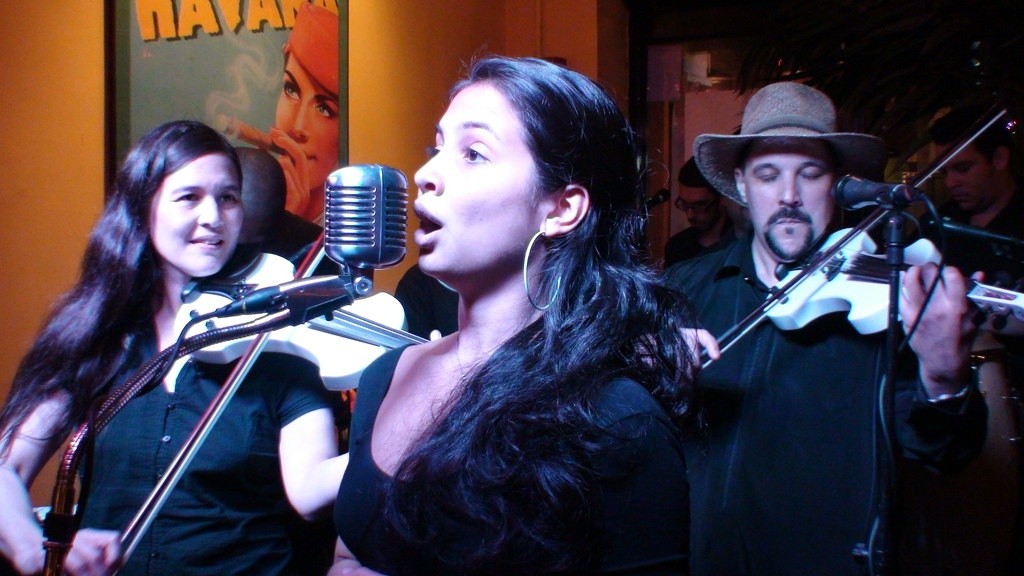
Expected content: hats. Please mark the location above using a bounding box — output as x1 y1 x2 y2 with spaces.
693 81 889 207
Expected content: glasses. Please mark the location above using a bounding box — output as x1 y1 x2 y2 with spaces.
673 197 709 210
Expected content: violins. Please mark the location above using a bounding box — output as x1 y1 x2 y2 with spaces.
168 247 438 396
758 222 1024 344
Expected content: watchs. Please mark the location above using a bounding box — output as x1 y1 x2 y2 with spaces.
918 379 972 414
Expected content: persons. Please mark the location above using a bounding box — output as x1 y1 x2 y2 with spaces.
633 80 993 576
0 119 350 576
330 55 700 576
662 104 1023 291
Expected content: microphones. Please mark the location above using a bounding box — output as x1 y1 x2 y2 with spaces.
832 173 923 212
216 162 409 319
